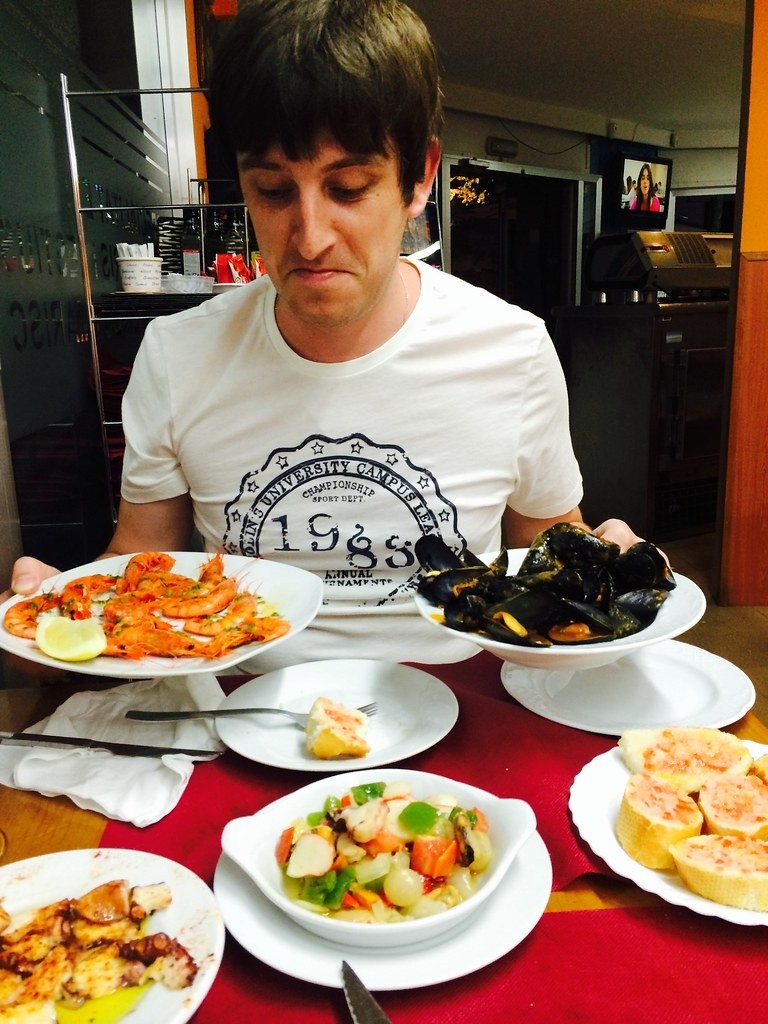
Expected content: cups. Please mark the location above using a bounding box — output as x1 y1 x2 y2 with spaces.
182 249 201 276
115 255 164 291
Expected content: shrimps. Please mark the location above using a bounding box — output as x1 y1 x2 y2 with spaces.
3 548 291 663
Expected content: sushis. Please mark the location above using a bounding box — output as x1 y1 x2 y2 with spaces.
615 727 768 910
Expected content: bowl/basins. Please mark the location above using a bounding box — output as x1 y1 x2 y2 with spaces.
221 768 538 949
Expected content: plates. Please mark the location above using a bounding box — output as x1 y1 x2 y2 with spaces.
0 848 226 1024
500 639 757 737
214 658 459 772
0 551 324 678
212 768 553 992
410 545 708 670
569 738 768 929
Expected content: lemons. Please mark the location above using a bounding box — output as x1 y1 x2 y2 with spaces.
37 616 105 661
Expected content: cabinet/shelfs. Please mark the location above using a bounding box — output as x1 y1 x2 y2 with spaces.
58 70 247 524
585 231 734 537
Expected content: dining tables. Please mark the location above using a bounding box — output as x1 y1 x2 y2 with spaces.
0 604 768 1024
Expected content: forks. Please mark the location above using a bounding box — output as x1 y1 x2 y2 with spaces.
123 701 378 729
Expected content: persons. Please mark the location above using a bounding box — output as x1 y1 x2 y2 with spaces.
621 163 664 212
0 0 672 679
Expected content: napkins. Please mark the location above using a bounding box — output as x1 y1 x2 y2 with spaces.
0 674 229 830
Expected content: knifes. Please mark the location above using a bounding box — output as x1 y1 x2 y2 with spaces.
341 961 392 1024
3 731 224 758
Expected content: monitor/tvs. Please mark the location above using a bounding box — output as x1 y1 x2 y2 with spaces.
598 147 673 231
